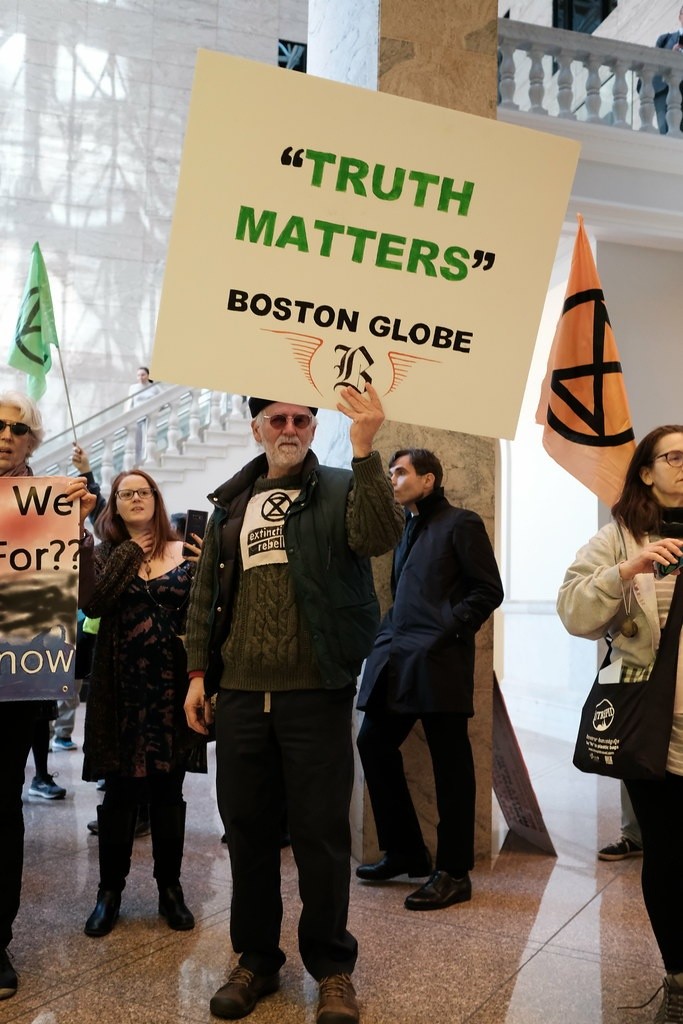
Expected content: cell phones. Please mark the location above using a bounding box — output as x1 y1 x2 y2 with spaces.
182 509 208 556
653 546 683 580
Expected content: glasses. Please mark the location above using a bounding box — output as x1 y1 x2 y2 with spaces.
0 419 33 436
115 487 156 500
263 414 312 428
654 450 683 467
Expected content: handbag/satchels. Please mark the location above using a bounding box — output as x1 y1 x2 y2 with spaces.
572 652 676 778
636 78 642 93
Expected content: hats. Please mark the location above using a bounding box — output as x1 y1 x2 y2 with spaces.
248 397 319 418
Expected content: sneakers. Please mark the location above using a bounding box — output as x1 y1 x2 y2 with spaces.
210 963 281 1020
617 974 683 1024
597 836 643 861
316 972 359 1024
28 772 66 799
52 736 78 750
0 947 21 997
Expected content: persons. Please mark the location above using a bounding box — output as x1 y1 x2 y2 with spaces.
597 779 643 860
123 367 161 412
0 390 97 999
652 3 683 137
28 445 215 839
356 449 503 911
78 471 208 938
183 397 402 1024
557 424 683 1024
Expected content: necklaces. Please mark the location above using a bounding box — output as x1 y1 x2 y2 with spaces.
143 540 159 574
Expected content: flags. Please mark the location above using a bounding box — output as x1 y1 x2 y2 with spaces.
9 246 59 402
536 228 634 508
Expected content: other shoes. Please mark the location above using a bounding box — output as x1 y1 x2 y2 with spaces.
133 817 150 838
87 818 99 835
95 779 106 791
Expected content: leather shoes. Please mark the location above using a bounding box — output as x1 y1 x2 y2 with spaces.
404 870 472 911
83 890 122 937
157 884 195 931
355 848 433 881
